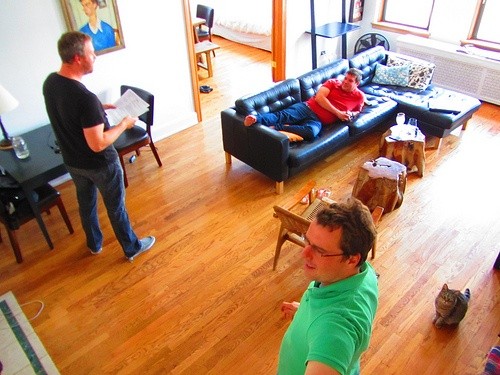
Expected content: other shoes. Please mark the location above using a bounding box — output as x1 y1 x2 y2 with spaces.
88 246 102 255
125 235 155 261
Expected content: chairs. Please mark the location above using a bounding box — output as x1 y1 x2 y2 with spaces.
196 4 216 57
0 183 74 263
273 180 384 271
110 85 162 187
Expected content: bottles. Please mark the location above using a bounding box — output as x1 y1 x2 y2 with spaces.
12 136 30 159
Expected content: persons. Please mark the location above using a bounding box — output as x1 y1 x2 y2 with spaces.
43 32 155 262
277 196 379 375
244 67 365 139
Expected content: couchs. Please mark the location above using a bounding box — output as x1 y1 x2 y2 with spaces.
221 46 481 194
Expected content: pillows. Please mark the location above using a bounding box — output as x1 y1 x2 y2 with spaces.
268 125 304 142
387 55 435 90
372 62 410 87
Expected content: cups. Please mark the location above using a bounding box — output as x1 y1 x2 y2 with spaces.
408 118 417 133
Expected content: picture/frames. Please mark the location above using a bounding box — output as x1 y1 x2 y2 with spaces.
59 0 125 56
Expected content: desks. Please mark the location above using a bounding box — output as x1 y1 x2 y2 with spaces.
379 125 426 178
0 122 68 250
351 156 407 214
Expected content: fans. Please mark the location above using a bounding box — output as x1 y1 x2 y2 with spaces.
353 33 390 55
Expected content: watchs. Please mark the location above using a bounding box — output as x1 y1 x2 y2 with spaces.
350 113 354 120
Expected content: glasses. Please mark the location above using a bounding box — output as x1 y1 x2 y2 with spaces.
300 231 346 258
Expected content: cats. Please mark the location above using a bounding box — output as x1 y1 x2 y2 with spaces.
431 283 471 329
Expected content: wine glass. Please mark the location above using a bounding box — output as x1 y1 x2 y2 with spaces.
396 112 405 127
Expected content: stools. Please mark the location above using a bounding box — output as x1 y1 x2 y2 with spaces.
195 40 220 77
